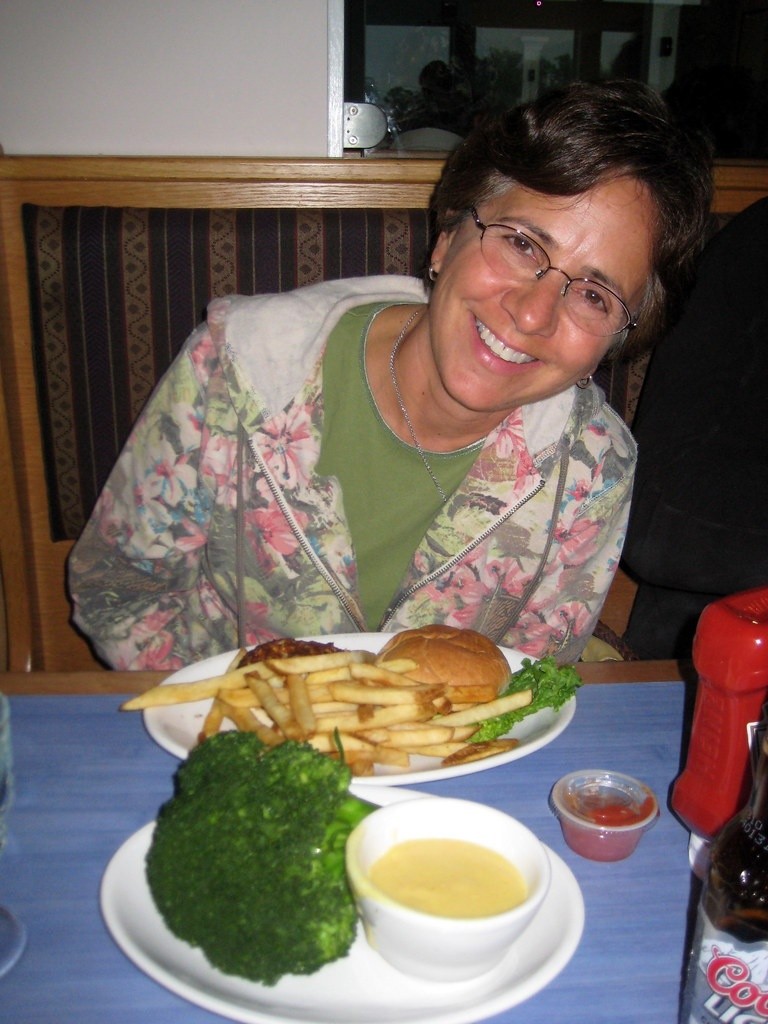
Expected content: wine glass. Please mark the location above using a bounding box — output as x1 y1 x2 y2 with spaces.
0 693 25 976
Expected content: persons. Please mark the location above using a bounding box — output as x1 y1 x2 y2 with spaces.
622 194 768 658
63 79 719 672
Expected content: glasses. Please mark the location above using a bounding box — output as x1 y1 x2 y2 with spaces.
471 204 639 336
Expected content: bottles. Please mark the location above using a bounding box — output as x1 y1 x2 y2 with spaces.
679 698 768 1023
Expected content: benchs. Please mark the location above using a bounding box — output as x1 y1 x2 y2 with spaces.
1 158 768 673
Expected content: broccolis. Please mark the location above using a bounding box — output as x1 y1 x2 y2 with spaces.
148 730 386 983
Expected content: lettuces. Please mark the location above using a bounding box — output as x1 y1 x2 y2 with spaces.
467 654 584 743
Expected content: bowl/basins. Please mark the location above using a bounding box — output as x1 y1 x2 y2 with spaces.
553 770 657 859
346 799 551 978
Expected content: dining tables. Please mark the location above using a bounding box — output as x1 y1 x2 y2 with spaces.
0 659 703 1024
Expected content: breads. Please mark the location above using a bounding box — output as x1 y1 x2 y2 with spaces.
371 624 509 704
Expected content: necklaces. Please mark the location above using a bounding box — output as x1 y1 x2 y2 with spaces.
389 304 447 504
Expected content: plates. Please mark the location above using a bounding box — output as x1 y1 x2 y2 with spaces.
97 781 587 1023
142 632 578 785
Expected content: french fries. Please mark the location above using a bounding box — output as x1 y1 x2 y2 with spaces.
116 647 534 774
